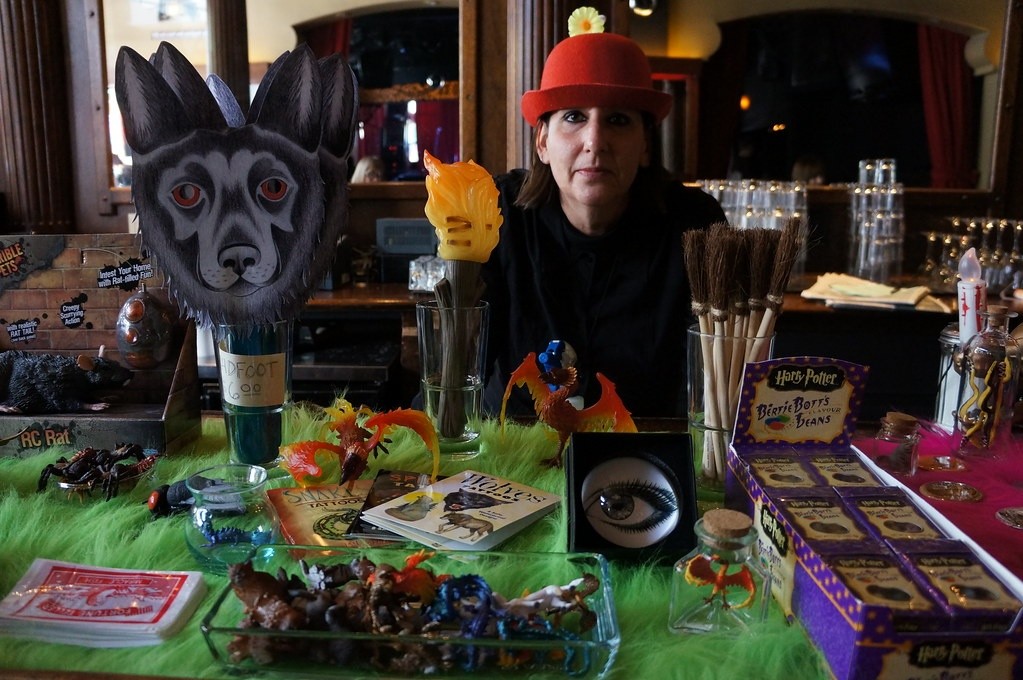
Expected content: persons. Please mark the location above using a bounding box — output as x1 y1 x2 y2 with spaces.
487 32 728 417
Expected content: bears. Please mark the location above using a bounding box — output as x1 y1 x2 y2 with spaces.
443 488 514 512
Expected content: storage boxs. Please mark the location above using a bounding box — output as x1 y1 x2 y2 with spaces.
723 359 1023 680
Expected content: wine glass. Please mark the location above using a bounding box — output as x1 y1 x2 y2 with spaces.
918 216 1023 293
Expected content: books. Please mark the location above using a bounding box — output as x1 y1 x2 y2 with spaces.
265 477 408 562
359 468 560 562
342 467 450 542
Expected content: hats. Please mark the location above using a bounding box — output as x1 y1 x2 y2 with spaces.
521 34 673 127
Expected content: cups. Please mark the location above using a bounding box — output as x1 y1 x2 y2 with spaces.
212 318 294 470
700 180 808 290
847 158 905 287
416 300 490 462
687 323 777 502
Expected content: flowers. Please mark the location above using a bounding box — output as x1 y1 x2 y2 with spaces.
567 5 607 37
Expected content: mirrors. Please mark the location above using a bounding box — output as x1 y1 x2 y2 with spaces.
610 1 1006 191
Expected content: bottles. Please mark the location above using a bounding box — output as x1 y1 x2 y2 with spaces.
954 306 1021 462
185 463 279 576
871 411 922 477
667 508 770 636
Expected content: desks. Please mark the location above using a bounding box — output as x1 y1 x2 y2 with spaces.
0 388 1023 680
301 274 958 404
